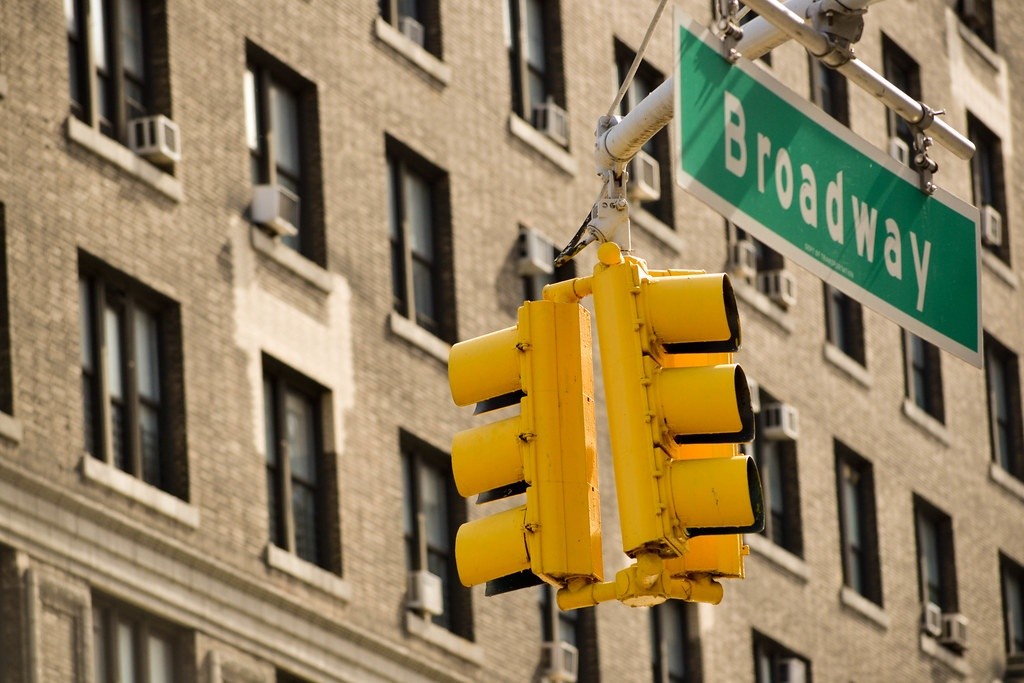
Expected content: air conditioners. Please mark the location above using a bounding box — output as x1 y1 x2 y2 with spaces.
918 595 943 641
940 613 969 652
515 227 556 280
731 241 758 281
534 99 572 144
891 136 911 166
744 372 762 416
397 15 425 48
403 570 444 616
761 395 798 440
253 183 304 236
977 200 1003 246
624 144 663 205
127 113 184 165
542 638 581 682
767 267 796 304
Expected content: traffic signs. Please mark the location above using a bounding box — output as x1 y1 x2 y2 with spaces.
669 12 986 369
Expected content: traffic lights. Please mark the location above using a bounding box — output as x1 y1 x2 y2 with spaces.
442 298 606 599
592 261 772 581
